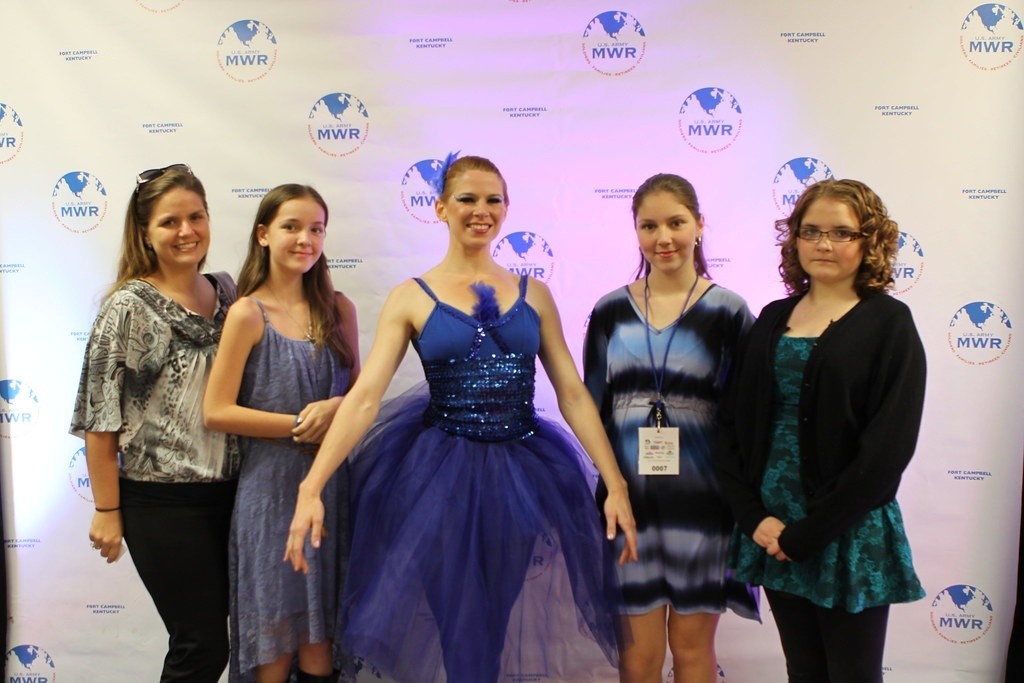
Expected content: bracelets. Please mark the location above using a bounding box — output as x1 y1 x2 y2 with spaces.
294 414 300 427
95 506 120 513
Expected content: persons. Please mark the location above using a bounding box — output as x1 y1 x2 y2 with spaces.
68 156 928 683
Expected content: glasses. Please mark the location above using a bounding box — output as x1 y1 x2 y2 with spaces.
795 226 863 242
135 164 187 205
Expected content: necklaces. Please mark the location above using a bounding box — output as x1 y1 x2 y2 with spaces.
262 282 316 343
